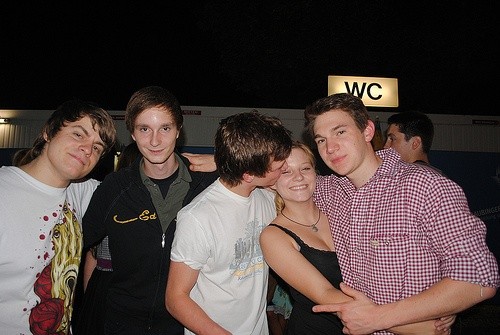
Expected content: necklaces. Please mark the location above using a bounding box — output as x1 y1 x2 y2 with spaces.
280 209 321 231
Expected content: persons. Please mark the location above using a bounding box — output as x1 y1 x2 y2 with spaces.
0 103 116 335
383 112 445 175
260 142 456 335
165 110 293 335
74 85 220 335
79 143 140 335
183 92 500 335
